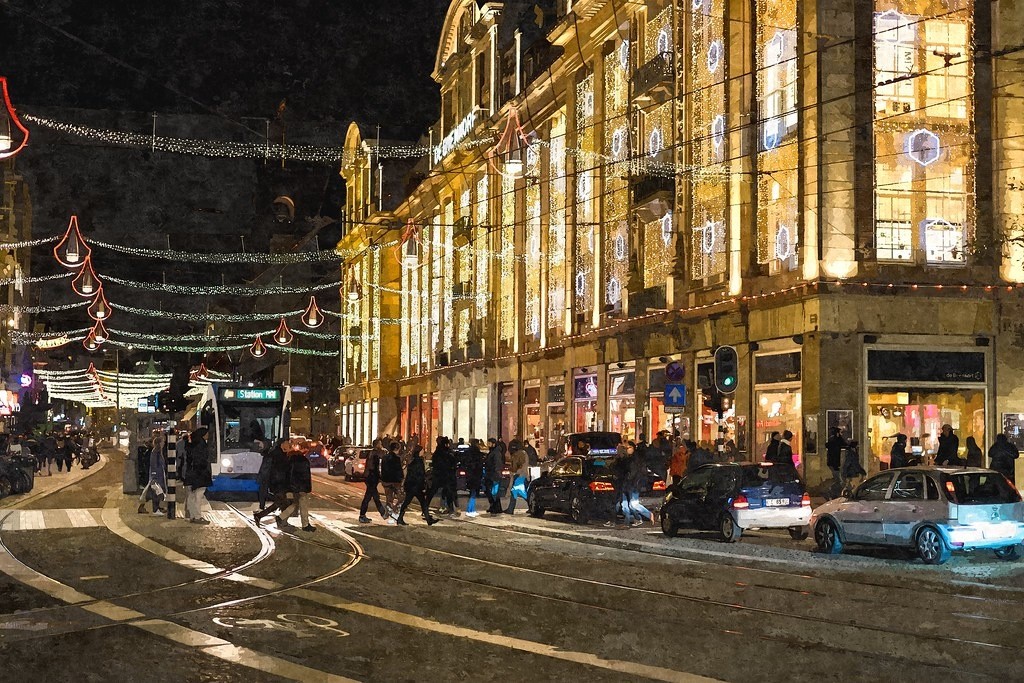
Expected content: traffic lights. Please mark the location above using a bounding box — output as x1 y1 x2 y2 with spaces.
713 345 739 394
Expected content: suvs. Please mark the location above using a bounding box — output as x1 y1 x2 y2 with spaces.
548 432 623 461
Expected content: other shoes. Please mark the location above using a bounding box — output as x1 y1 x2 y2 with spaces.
504 510 514 515
253 514 260 528
632 519 643 527
397 518 409 525
359 516 372 523
138 507 149 514
618 525 630 530
455 507 462 517
466 510 479 518
486 508 497 512
190 516 210 524
302 524 317 531
153 510 164 517
603 521 615 528
526 508 532 512
383 513 392 520
650 514 654 526
428 518 439 526
275 515 282 528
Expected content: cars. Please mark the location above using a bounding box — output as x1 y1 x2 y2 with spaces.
344 448 382 483
451 447 510 499
660 461 813 543
289 433 329 468
526 452 667 526
810 464 1024 565
328 445 364 476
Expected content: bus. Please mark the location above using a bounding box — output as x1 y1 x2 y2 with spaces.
195 385 293 495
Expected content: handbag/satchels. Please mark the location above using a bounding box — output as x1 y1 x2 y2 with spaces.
142 480 167 502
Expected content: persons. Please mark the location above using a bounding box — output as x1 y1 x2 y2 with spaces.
824 428 866 502
319 433 352 454
12 431 96 476
253 438 317 533
358 433 537 526
137 436 167 516
934 424 982 468
890 434 908 469
603 440 654 529
637 429 746 484
186 427 213 525
988 434 1020 486
762 431 796 482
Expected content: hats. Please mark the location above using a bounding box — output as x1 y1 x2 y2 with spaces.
782 429 792 440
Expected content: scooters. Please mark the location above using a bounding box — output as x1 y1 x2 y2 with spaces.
79 446 101 469
0 447 37 499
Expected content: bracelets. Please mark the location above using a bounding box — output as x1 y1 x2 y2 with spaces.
946 459 949 462
